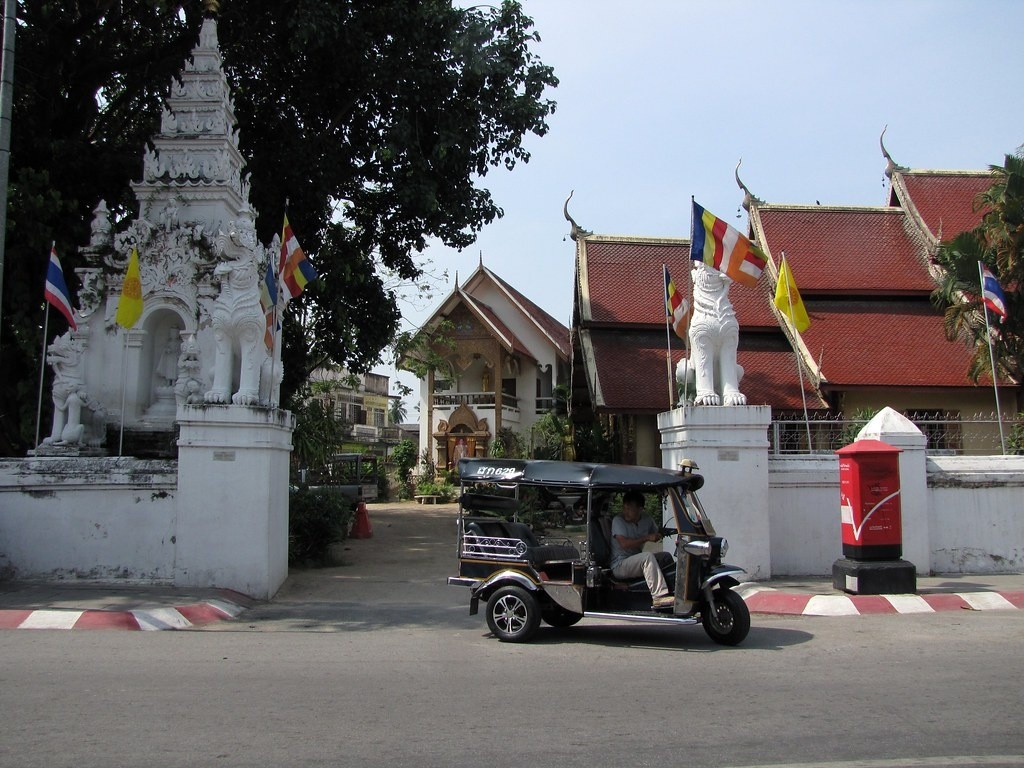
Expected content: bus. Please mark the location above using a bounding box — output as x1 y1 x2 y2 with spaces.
289 453 379 511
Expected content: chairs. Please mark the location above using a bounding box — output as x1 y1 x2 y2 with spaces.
593 514 623 582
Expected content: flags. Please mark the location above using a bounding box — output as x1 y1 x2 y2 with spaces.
691 200 767 290
116 248 144 330
776 260 809 333
258 210 317 350
979 265 1008 325
663 264 691 343
44 247 78 331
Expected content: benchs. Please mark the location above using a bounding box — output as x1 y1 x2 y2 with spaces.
460 494 582 578
414 495 440 504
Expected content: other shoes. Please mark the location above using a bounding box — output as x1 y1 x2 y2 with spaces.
653 596 675 608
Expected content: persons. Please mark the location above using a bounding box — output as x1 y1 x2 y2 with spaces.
453 439 467 467
610 494 676 607
155 326 184 388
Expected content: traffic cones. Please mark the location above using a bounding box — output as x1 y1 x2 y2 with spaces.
349 502 374 539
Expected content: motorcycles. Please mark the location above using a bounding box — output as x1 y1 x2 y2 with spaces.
447 458 750 646
